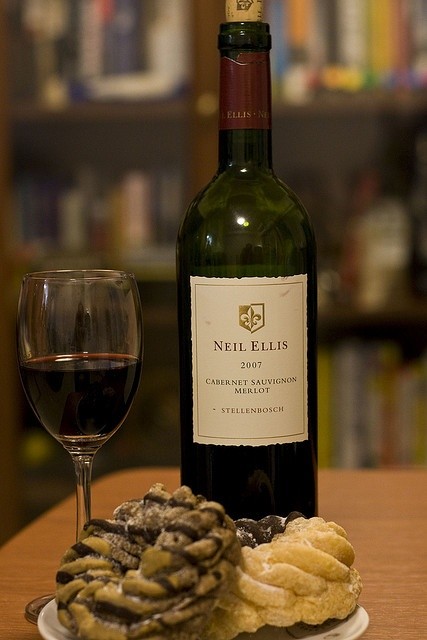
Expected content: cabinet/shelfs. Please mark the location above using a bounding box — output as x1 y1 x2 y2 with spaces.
1 1 427 546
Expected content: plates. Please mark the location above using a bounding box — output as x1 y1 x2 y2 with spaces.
38 600 370 640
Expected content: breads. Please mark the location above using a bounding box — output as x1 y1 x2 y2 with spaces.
55 483 239 638
205 511 362 640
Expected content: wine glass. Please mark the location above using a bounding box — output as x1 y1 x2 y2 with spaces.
14 269 146 625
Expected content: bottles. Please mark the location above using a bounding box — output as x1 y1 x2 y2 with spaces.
177 2 317 520
